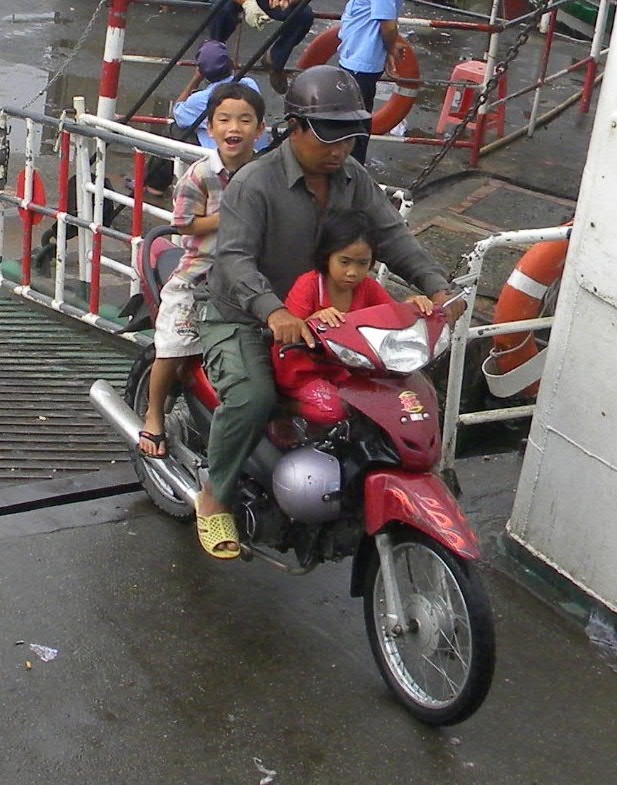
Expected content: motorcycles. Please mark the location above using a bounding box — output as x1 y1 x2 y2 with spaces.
89 225 496 727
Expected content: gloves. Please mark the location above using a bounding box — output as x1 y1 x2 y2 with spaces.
241 0 275 31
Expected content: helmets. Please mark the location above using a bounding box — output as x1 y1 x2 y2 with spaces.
272 441 341 524
283 64 371 144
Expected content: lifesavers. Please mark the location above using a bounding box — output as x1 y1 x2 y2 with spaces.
493 219 574 400
298 24 420 137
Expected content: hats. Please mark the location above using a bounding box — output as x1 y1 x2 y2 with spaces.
306 117 371 143
196 39 232 81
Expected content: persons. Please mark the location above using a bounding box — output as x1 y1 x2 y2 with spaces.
144 39 271 196
206 0 316 94
191 66 465 559
273 210 435 440
335 0 410 167
137 83 265 461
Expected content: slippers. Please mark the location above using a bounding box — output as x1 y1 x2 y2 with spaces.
195 490 241 558
126 179 165 199
136 430 169 459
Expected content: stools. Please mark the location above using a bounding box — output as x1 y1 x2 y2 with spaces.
436 60 507 144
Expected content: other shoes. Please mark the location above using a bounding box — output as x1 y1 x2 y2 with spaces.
261 50 289 93
